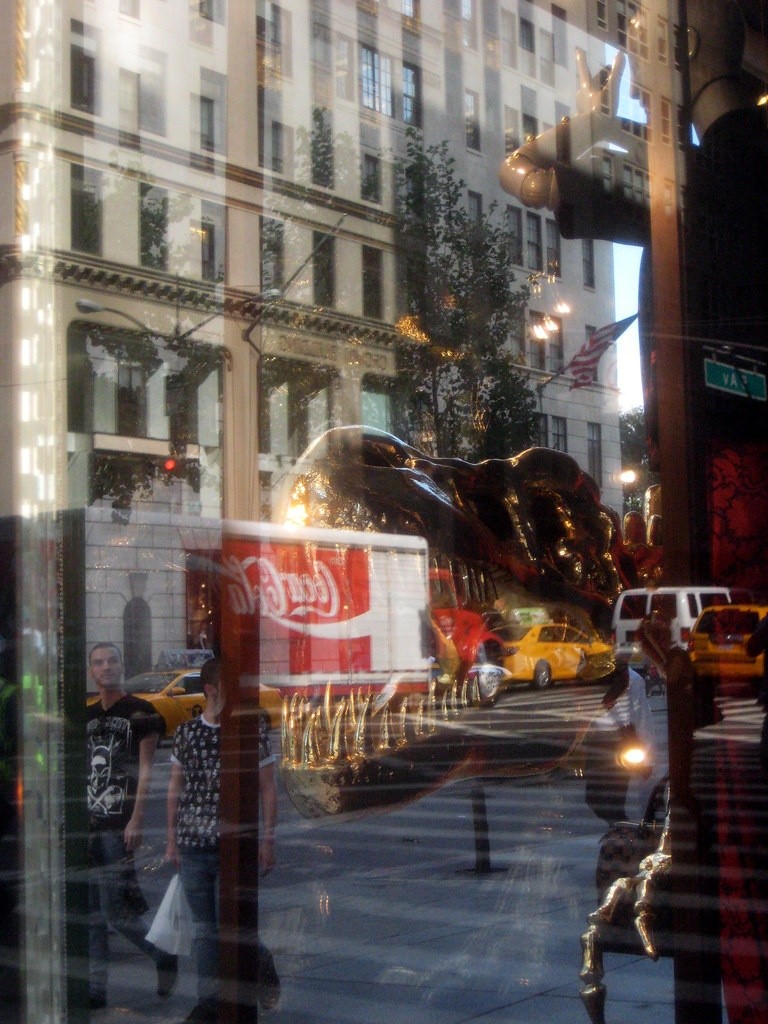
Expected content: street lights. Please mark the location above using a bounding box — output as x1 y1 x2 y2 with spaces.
76 288 284 516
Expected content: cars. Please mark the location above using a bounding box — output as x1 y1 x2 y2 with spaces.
686 603 768 688
430 566 505 712
487 621 615 691
87 666 288 748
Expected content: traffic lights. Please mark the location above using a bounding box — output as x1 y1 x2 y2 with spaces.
165 457 184 474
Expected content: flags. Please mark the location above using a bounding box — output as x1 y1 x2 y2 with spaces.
570 315 639 390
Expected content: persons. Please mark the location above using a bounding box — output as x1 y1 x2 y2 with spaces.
83 609 719 1024
499 0 768 471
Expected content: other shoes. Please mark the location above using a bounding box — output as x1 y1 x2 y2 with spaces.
157 954 179 999
258 950 281 1011
89 991 107 1008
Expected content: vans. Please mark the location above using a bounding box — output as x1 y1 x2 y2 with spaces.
609 585 766 691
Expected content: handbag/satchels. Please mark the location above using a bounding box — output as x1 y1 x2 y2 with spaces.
145 874 198 957
